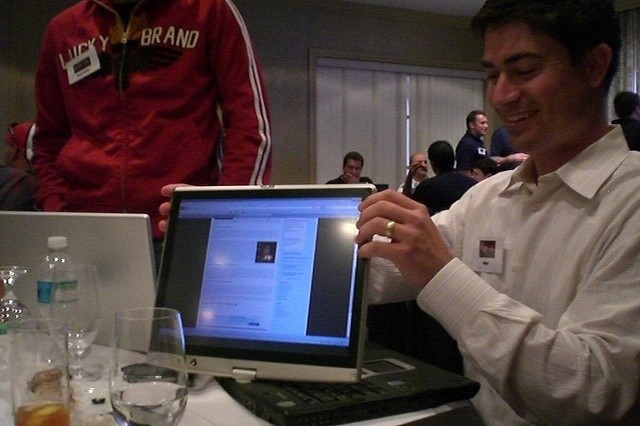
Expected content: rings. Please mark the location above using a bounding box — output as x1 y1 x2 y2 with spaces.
386 219 397 236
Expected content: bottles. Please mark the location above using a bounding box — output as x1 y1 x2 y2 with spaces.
36 237 80 335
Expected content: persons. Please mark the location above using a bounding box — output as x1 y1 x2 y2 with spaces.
611 91 639 150
34 1 273 261
395 153 432 195
492 125 521 154
257 241 274 262
411 141 478 216
472 168 489 180
158 1 639 424
325 150 371 183
455 110 530 172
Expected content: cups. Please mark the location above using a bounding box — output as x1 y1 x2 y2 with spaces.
6 319 70 426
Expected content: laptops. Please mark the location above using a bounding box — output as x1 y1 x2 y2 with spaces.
0 209 160 355
147 184 481 425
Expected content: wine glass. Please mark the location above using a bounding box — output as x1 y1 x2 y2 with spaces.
1 264 34 370
48 263 103 383
108 308 188 426
